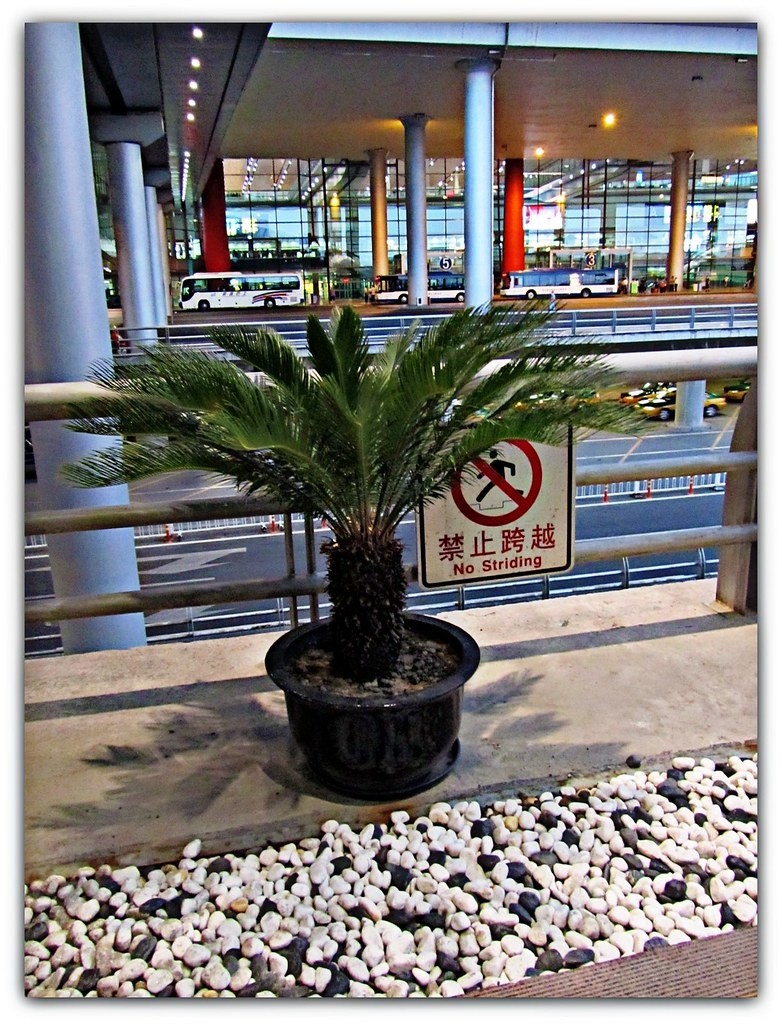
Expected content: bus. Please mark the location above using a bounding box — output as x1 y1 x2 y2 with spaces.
178 271 304 311
499 267 618 300
374 270 464 303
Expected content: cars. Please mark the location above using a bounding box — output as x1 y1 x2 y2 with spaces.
621 382 727 421
516 388 600 412
724 376 754 402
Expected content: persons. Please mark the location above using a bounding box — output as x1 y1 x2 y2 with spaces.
548 289 556 311
330 286 335 304
364 285 376 303
745 278 752 289
724 274 730 288
618 275 678 294
702 275 710 292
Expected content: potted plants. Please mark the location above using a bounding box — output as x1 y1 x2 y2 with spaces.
59 295 657 802
